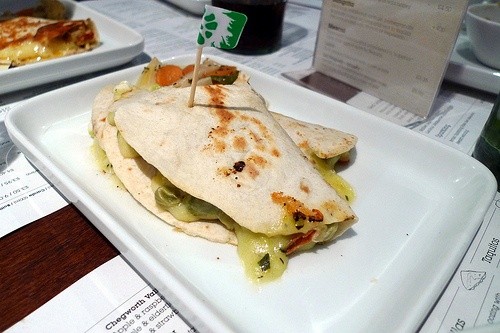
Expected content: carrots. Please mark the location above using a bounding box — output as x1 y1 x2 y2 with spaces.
156 64 237 86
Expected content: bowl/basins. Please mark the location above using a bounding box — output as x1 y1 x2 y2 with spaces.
466 3 500 68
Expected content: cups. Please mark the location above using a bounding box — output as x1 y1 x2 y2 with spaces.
211 0 286 55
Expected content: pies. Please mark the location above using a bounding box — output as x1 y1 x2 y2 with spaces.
90 80 359 253
0 15 99 64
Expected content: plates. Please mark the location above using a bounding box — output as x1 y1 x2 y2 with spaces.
4 53 497 332
0 0 144 94
